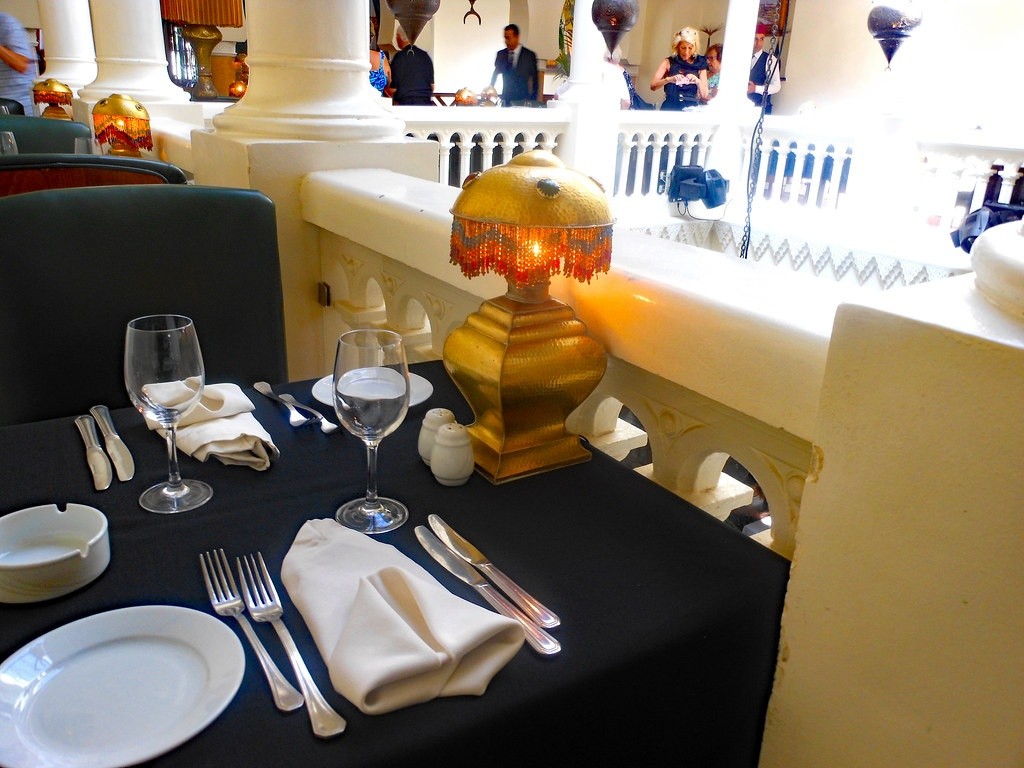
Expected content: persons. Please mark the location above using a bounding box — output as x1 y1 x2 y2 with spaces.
605 44 634 110
390 25 434 106
746 23 781 114
649 27 709 111
602 61 630 111
490 23 538 107
369 32 392 97
696 43 723 106
0 13 38 118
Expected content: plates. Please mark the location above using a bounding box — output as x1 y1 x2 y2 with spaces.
1 603 247 768
310 365 434 411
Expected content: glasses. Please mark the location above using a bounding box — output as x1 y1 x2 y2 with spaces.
707 56 718 62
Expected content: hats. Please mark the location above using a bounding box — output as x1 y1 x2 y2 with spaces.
756 24 766 36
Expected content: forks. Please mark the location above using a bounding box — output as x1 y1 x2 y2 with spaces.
253 380 325 428
236 552 347 741
199 547 307 713
278 393 345 435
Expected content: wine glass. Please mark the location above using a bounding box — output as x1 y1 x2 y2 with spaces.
123 314 214 516
331 329 409 534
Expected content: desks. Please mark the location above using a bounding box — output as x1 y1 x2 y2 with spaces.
0 361 791 768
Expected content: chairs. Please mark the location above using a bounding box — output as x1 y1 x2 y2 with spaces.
0 114 92 154
0 152 188 197
0 183 289 429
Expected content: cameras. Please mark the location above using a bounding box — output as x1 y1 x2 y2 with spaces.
668 165 730 209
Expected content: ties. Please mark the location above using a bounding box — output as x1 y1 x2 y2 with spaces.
508 52 514 67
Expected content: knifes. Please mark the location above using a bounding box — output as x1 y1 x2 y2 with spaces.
427 511 561 631
74 413 112 491
413 527 564 657
90 402 134 483
